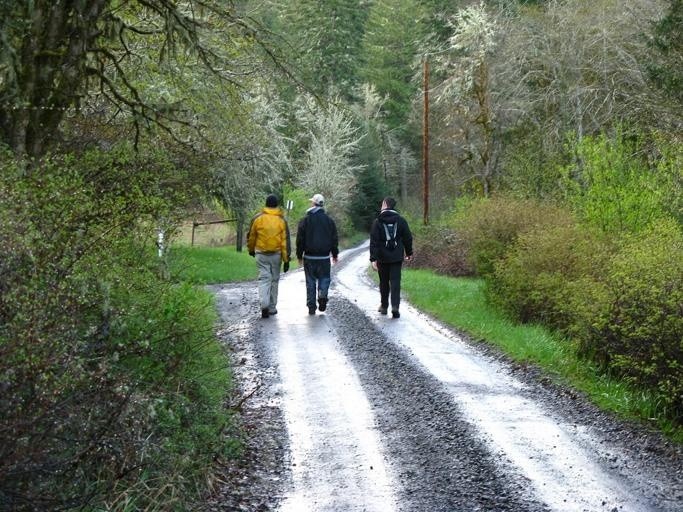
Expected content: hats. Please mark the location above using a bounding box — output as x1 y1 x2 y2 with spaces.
309 194 324 205
266 195 276 207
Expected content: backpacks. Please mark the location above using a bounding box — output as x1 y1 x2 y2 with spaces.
377 218 399 250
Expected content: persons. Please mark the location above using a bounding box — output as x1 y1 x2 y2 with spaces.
245 194 291 318
369 196 413 319
295 193 338 314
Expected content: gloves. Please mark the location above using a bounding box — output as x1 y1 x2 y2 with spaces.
284 262 289 272
249 251 255 255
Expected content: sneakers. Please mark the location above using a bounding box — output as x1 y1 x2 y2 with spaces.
308 305 315 313
262 308 277 318
318 298 326 311
392 306 399 318
378 305 387 313
